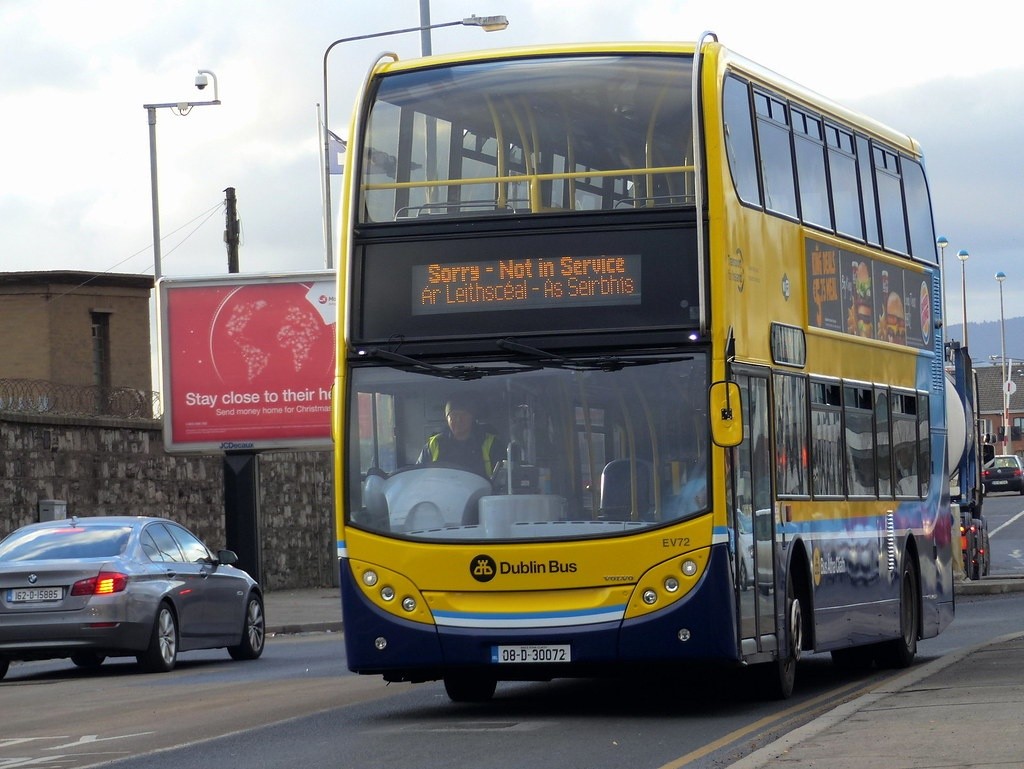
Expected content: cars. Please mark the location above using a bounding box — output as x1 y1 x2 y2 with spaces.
982 454 1024 497
0 515 265 673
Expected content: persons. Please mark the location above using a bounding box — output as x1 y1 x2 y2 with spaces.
417 396 507 483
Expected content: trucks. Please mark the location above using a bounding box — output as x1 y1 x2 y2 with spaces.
939 340 990 586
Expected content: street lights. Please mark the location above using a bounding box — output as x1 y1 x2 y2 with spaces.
956 250 971 348
144 69 222 283
992 272 1013 460
935 235 947 337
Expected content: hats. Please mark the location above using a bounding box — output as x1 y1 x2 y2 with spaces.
445 392 474 418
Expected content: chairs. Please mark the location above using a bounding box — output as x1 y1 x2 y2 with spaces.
598 456 651 523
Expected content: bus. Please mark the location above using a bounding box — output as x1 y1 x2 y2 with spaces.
329 28 983 719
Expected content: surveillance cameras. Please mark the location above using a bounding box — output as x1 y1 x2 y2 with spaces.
195 75 208 90
